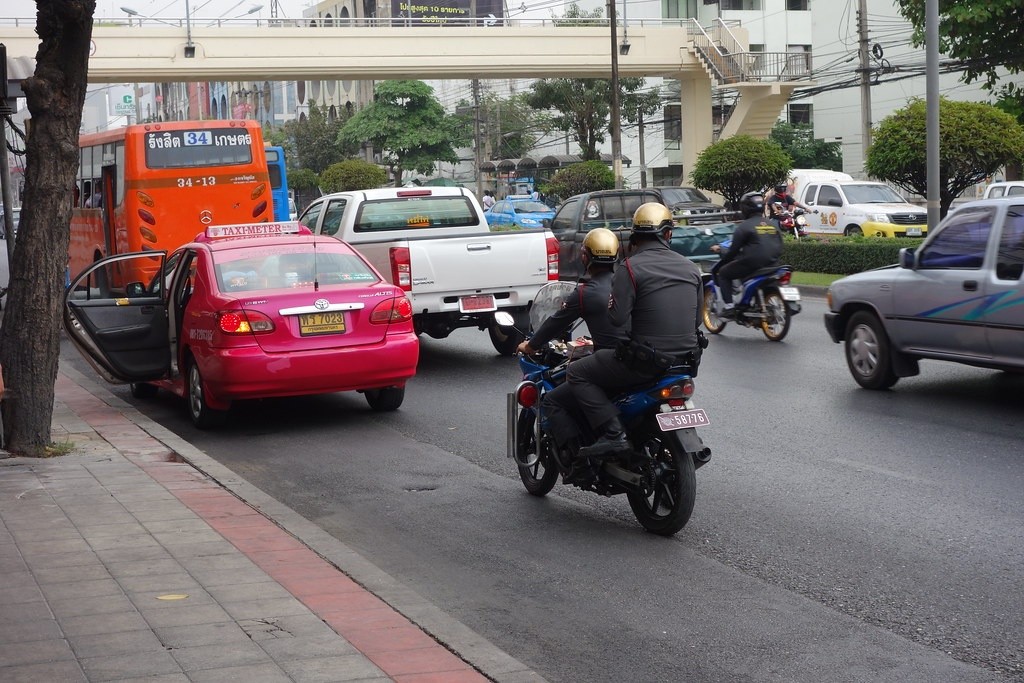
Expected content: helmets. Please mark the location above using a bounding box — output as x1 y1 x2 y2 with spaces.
628 202 674 245
738 192 765 217
583 228 620 264
774 181 787 193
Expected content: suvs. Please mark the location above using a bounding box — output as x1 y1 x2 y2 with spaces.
657 185 728 223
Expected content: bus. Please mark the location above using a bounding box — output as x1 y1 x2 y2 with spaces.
16 119 274 298
262 145 291 223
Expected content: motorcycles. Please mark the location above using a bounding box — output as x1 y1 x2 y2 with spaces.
493 281 716 536
702 239 802 341
774 209 820 240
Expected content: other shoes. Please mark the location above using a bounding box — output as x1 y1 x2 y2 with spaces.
579 430 634 458
716 307 736 317
562 461 588 484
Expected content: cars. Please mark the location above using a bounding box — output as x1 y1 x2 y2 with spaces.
822 193 1024 389
288 198 297 222
483 194 558 230
0 203 21 239
61 219 420 429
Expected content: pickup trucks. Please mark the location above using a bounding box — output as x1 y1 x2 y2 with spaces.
541 187 746 282
946 180 1024 217
782 168 928 242
292 186 560 357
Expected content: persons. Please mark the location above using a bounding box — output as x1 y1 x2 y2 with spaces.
85 181 103 209
711 191 784 317
483 190 495 211
768 186 813 232
527 186 545 202
516 202 705 490
